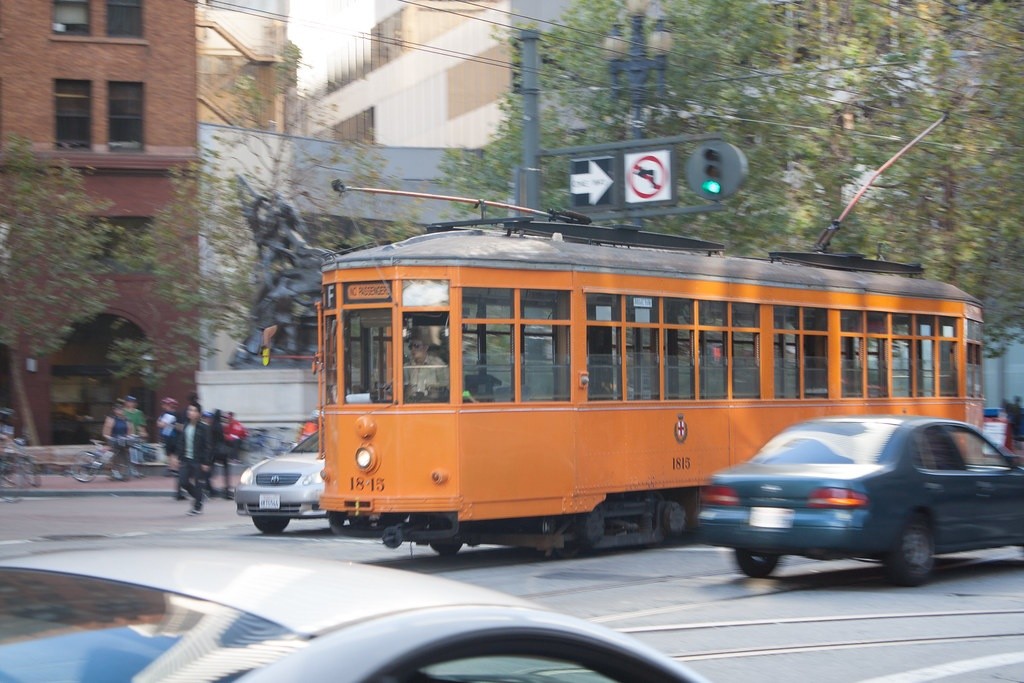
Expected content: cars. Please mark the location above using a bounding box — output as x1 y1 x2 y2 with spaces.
234 425 327 534
696 417 1024 588
0 538 710 683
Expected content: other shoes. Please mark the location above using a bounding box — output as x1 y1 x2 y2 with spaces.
128 469 144 478
111 470 122 481
191 502 201 514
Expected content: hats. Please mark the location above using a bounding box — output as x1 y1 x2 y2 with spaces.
126 395 135 403
162 398 177 407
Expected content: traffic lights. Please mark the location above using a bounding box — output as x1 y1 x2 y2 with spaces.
702 149 720 194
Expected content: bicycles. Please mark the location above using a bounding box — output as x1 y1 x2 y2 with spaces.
0 433 40 503
65 433 158 483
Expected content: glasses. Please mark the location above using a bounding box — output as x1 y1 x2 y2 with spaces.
408 344 423 350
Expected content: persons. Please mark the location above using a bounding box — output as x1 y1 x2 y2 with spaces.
297 409 325 446
1001 396 1022 443
403 328 449 396
175 402 215 516
156 391 247 500
102 395 148 481
0 408 25 456
237 190 323 355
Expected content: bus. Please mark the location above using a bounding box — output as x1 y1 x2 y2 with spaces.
263 211 985 557
